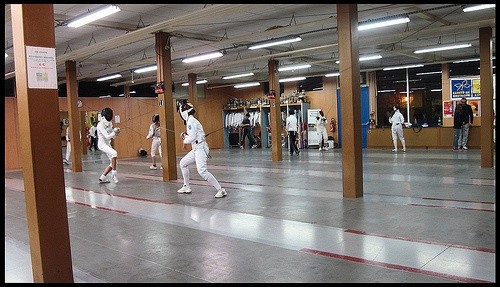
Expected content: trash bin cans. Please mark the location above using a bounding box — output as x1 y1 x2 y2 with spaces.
327 136 336 149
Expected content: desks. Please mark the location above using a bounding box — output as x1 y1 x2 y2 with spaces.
222 102 309 151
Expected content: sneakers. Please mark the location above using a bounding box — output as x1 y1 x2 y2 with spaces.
99 178 110 183
160 167 163 170
176 185 192 193
112 174 120 184
150 165 158 169
214 188 227 198
64 159 69 165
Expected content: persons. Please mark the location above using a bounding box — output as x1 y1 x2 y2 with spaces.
452 99 474 151
97 108 121 184
425 98 441 126
145 114 164 170
286 110 300 157
388 105 406 152
239 113 256 149
63 126 71 165
89 121 100 151
177 102 228 198
316 111 329 151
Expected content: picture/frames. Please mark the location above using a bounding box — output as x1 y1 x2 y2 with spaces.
97 113 102 122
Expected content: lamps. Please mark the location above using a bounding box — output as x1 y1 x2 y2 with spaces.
133 65 157 74
278 61 311 82
461 4 496 12
358 15 410 31
181 79 207 87
325 41 495 77
221 72 260 89
247 34 303 50
181 50 223 64
65 4 121 28
96 73 122 81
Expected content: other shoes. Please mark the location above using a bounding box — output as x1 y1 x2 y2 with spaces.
400 148 406 152
240 146 244 150
453 148 462 151
88 149 92 151
392 148 398 152
463 147 468 150
323 146 329 150
252 145 257 149
319 147 322 151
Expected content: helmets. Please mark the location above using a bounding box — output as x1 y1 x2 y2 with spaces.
179 103 195 120
152 114 160 122
101 108 113 124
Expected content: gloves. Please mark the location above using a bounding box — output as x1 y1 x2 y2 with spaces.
179 132 187 139
